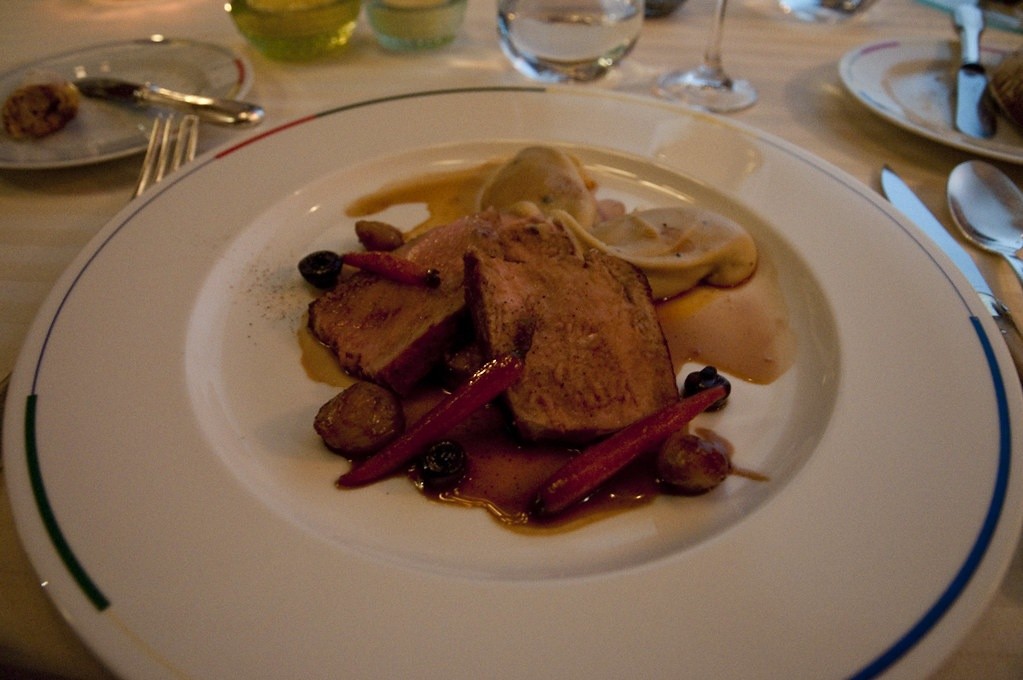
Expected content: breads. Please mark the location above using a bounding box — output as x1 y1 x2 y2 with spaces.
463 220 681 440
307 204 522 396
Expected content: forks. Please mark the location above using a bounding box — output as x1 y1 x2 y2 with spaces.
129 115 199 202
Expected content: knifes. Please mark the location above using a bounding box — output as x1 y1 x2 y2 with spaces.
879 167 1023 377
952 4 998 139
77 74 265 129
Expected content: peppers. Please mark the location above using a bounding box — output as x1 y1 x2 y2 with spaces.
536 384 725 516
336 350 523 486
336 252 441 288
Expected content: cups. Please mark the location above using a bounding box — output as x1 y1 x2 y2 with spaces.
495 0 643 85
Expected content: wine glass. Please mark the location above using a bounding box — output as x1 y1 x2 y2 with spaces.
651 0 759 113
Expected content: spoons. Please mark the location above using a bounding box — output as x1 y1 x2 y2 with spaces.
945 159 1023 286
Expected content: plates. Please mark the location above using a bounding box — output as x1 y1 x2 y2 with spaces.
0 35 255 168
838 36 1023 163
3 83 1023 680
